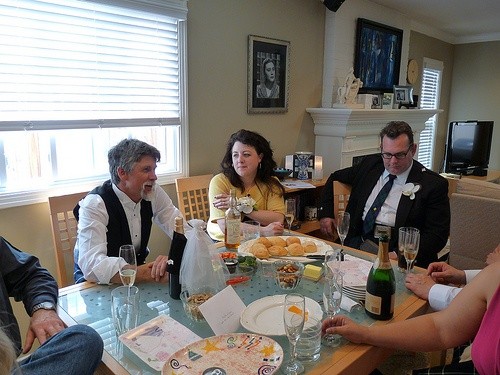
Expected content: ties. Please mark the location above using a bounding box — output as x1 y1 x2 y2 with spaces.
360 174 397 242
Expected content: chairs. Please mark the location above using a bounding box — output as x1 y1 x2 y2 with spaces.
175 174 213 222
49 190 90 288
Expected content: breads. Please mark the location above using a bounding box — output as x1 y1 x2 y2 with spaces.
249 236 317 259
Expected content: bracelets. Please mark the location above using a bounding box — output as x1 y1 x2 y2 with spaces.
236 197 257 214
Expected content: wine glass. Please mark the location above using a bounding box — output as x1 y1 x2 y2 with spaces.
284 199 350 249
404 232 420 275
279 272 344 375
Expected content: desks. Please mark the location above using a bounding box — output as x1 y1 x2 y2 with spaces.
57 229 441 375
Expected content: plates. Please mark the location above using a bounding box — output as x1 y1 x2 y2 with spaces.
333 275 367 301
118 294 323 374
237 237 333 263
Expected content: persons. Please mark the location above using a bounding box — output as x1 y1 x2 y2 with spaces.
397 93 401 100
321 261 500 375
72 140 189 284
0 235 104 375
344 68 356 99
371 97 379 106
256 58 279 98
403 262 482 311
319 121 450 267
206 130 288 244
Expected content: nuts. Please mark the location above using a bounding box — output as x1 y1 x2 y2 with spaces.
186 292 212 320
275 264 302 288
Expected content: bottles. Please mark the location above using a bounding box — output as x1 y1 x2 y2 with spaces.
365 234 396 321
224 188 242 249
168 215 187 299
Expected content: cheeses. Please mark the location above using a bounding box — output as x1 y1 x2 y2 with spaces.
304 264 322 278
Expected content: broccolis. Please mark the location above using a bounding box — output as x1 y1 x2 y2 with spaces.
237 255 259 271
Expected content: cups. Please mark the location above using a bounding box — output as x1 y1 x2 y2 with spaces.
112 244 139 337
295 315 322 361
396 226 421 272
325 250 342 273
242 220 260 240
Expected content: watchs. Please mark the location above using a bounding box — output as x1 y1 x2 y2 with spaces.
33 302 56 312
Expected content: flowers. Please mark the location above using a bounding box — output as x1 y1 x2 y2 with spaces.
401 182 420 200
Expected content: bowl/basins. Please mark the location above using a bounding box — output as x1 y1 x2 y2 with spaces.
215 258 305 291
273 168 293 181
181 286 222 321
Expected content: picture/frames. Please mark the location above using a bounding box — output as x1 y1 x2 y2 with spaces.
393 85 414 105
355 18 404 94
372 94 382 108
246 34 290 114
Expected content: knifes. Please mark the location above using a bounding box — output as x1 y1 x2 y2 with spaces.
269 254 325 259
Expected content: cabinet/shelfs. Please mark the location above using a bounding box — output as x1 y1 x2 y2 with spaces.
281 178 328 233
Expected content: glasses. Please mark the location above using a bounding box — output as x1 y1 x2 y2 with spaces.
381 143 414 159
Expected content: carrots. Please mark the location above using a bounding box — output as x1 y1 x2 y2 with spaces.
218 252 237 259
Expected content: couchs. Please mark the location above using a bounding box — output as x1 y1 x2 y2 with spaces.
449 177 500 271
